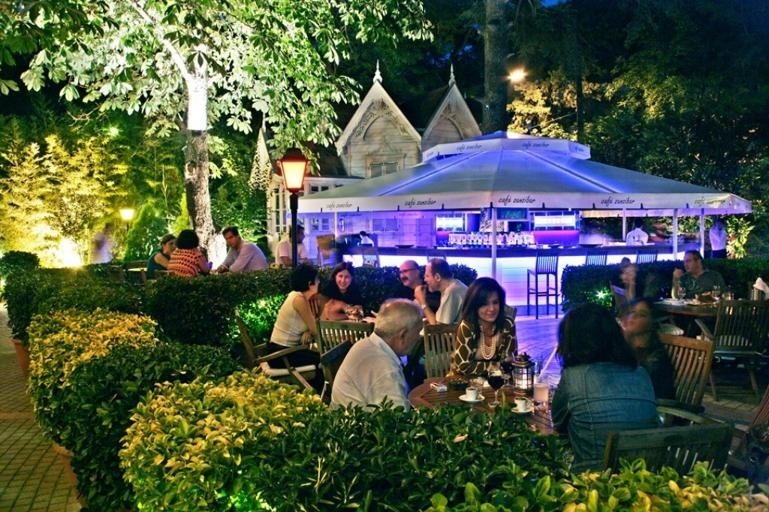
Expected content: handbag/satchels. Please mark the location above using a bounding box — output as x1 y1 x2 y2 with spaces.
726 428 769 482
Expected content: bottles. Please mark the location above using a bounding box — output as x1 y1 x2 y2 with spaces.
726 284 734 301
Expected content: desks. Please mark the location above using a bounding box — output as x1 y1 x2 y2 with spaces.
410 376 560 437
652 300 757 342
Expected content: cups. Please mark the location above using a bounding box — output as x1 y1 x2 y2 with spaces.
534 374 549 410
465 386 478 399
513 396 528 410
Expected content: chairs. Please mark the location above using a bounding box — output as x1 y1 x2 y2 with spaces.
650 334 712 411
235 316 319 392
310 292 324 315
698 299 769 394
316 318 376 379
605 408 734 474
525 247 661 320
423 324 460 378
427 253 447 265
318 339 353 414
104 247 379 287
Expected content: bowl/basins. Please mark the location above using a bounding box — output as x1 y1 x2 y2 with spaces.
448 382 469 390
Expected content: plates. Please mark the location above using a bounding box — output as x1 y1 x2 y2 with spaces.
458 394 485 403
510 405 533 414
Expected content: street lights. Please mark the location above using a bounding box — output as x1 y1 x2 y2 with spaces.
268 142 314 273
119 204 139 233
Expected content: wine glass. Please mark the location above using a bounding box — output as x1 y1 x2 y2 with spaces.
678 287 686 303
486 364 505 407
500 351 516 387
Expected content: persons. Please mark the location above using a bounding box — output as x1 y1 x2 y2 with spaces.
670 250 728 301
626 220 650 247
709 221 728 258
266 258 365 382
146 226 307 280
329 298 423 413
360 231 374 246
445 277 518 377
550 296 676 469
398 258 469 325
